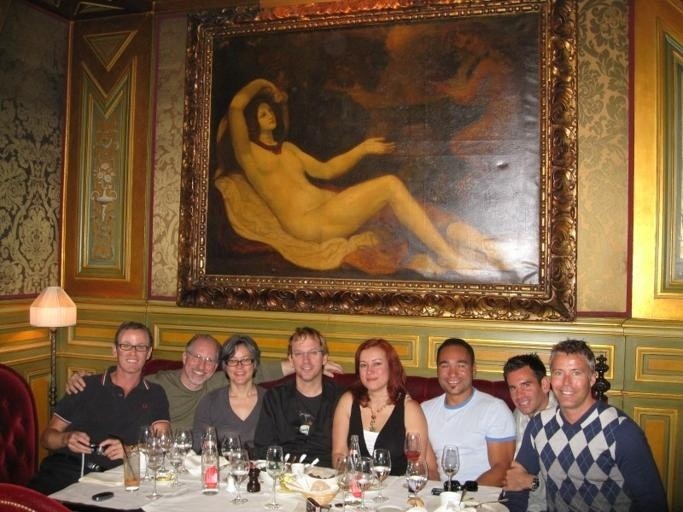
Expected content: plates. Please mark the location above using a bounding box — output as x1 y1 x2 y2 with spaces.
460 500 510 512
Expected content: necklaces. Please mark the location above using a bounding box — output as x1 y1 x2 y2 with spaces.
366 401 388 431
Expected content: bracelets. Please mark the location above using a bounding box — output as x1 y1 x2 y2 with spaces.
531 477 539 491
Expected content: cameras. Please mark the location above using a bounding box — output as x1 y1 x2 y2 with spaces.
89 440 105 457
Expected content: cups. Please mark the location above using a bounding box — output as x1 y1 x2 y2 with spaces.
123 445 140 491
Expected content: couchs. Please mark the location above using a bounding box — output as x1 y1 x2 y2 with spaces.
140 359 610 510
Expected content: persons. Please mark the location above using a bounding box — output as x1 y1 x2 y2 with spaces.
40 321 171 512
503 352 559 511
193 335 270 458
228 78 480 269
420 337 517 486
254 327 350 469
332 339 428 476
498 337 671 512
65 334 344 438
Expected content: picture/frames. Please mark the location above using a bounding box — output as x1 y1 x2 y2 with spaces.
623 0 683 322
175 0 580 323
58 10 158 305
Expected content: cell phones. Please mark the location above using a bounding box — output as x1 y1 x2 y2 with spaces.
92 492 113 501
432 488 443 494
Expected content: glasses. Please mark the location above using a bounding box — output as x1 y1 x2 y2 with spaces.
187 350 213 363
444 480 478 492
116 343 149 352
225 359 254 366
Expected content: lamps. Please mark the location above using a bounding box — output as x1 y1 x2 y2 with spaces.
29 286 78 424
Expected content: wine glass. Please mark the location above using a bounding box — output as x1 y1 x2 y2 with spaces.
139 426 460 512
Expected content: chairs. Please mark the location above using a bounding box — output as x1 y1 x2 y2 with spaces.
0 364 70 512
